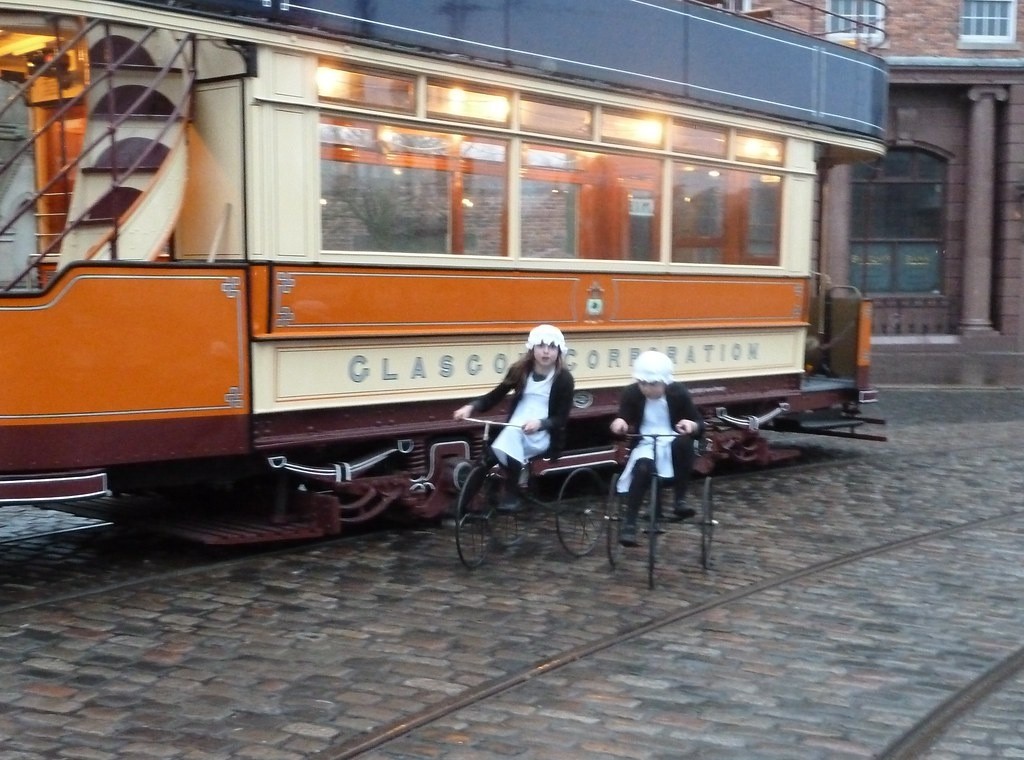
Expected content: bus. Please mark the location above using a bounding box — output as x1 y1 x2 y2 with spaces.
0 0 896 554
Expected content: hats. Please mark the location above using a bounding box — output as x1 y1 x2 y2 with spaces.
633 351 674 384
528 325 565 353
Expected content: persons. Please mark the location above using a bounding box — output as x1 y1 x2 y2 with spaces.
452 324 573 525
613 349 707 545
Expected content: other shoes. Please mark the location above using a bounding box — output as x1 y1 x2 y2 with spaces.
498 498 519 510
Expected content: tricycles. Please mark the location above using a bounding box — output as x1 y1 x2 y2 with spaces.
453 415 609 571
603 432 718 592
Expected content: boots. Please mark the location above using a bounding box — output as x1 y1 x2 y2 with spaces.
620 492 642 545
673 481 696 517
